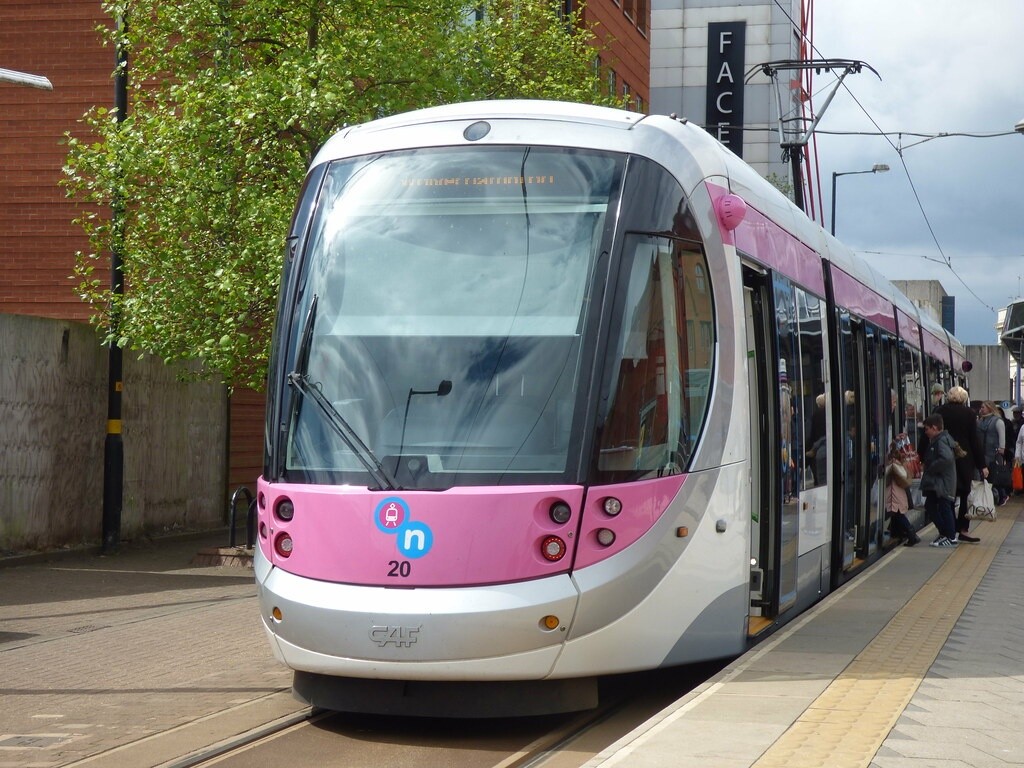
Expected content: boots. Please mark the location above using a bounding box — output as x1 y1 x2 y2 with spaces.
903 527 920 546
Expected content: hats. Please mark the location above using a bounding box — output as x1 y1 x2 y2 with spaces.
932 383 944 394
1011 407 1023 412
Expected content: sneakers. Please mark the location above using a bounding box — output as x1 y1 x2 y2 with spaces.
930 534 944 546
997 495 1009 506
934 536 958 547
845 528 855 540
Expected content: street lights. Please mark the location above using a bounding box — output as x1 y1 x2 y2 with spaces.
831 163 890 237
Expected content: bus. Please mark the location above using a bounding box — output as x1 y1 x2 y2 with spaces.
250 96 975 721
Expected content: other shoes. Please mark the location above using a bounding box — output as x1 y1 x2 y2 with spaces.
959 534 980 544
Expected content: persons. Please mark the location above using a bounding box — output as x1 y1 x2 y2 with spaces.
931 383 989 544
886 388 921 547
781 388 877 541
969 400 1024 508
918 414 958 547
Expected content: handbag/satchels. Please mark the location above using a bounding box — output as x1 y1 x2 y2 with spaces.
990 453 1011 488
801 469 815 490
1011 460 1023 490
902 435 921 479
965 479 997 522
